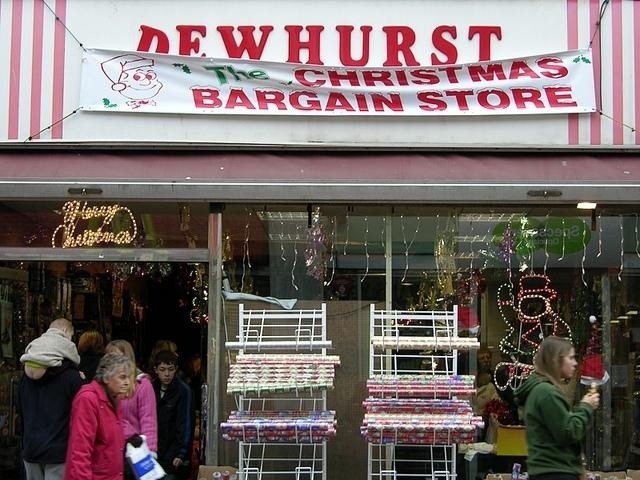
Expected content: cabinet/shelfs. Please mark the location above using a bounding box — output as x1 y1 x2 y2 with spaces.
228 303 330 480
366 304 460 480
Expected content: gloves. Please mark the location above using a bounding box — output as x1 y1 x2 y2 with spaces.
129 434 144 448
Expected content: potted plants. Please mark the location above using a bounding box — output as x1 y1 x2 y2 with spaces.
485 368 531 457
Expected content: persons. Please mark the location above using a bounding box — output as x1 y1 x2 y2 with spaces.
152 350 196 480
75 331 105 380
478 348 512 403
18 319 80 381
512 334 600 480
473 371 501 412
104 340 158 453
14 367 90 480
63 352 133 480
148 339 179 373
